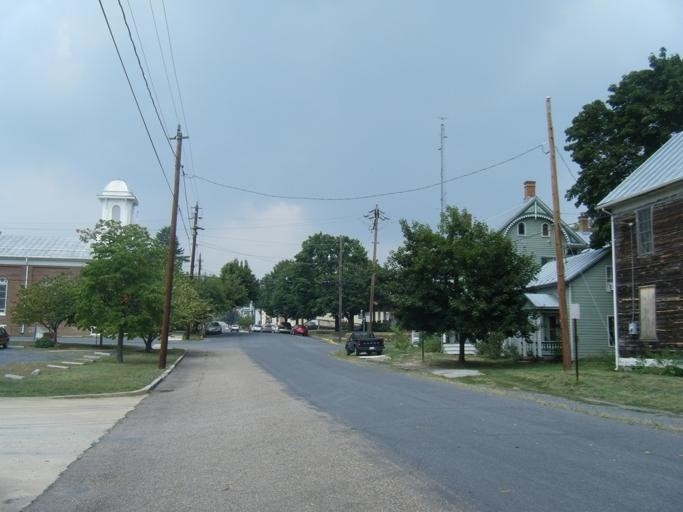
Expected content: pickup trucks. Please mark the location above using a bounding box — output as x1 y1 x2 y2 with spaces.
344 329 384 356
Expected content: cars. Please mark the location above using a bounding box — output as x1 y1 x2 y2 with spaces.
204 320 222 336
0 326 10 349
230 324 241 334
247 321 319 337
